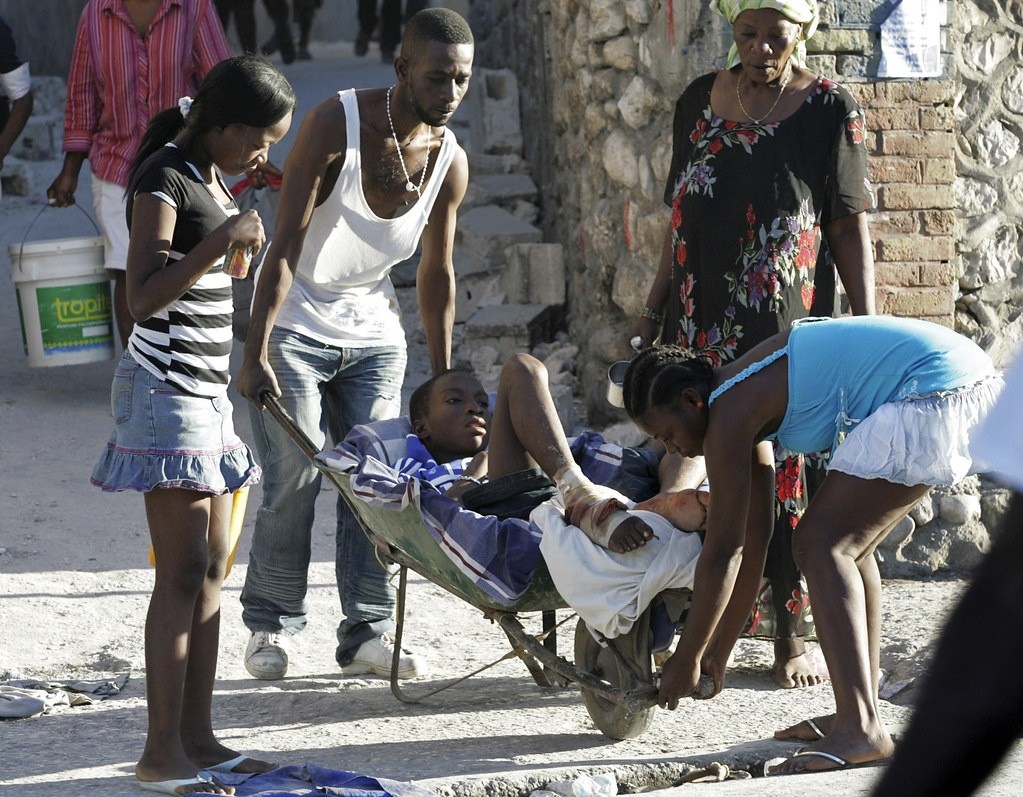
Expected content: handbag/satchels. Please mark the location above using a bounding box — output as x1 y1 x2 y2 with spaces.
215 173 285 342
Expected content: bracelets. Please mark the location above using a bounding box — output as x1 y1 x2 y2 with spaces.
454 475 480 485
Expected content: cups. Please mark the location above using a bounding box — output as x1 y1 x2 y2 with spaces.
603 357 631 408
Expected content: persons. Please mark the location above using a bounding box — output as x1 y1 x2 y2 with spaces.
45 0 231 352
90 52 296 796
211 0 431 64
0 16 33 172
409 350 712 554
234 5 477 681
622 313 1008 777
865 344 1023 797
627 0 879 690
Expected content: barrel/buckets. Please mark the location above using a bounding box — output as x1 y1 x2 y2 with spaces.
7 197 115 369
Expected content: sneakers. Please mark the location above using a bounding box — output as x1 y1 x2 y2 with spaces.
341 633 418 679
244 630 287 680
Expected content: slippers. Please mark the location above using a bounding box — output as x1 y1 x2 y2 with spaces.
774 718 828 744
138 771 235 797
766 746 896 777
200 754 274 786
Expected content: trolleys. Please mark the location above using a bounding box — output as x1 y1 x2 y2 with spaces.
256 364 718 745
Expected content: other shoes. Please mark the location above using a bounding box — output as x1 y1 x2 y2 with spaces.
242 24 399 65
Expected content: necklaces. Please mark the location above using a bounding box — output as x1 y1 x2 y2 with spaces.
736 59 794 124
386 83 432 225
